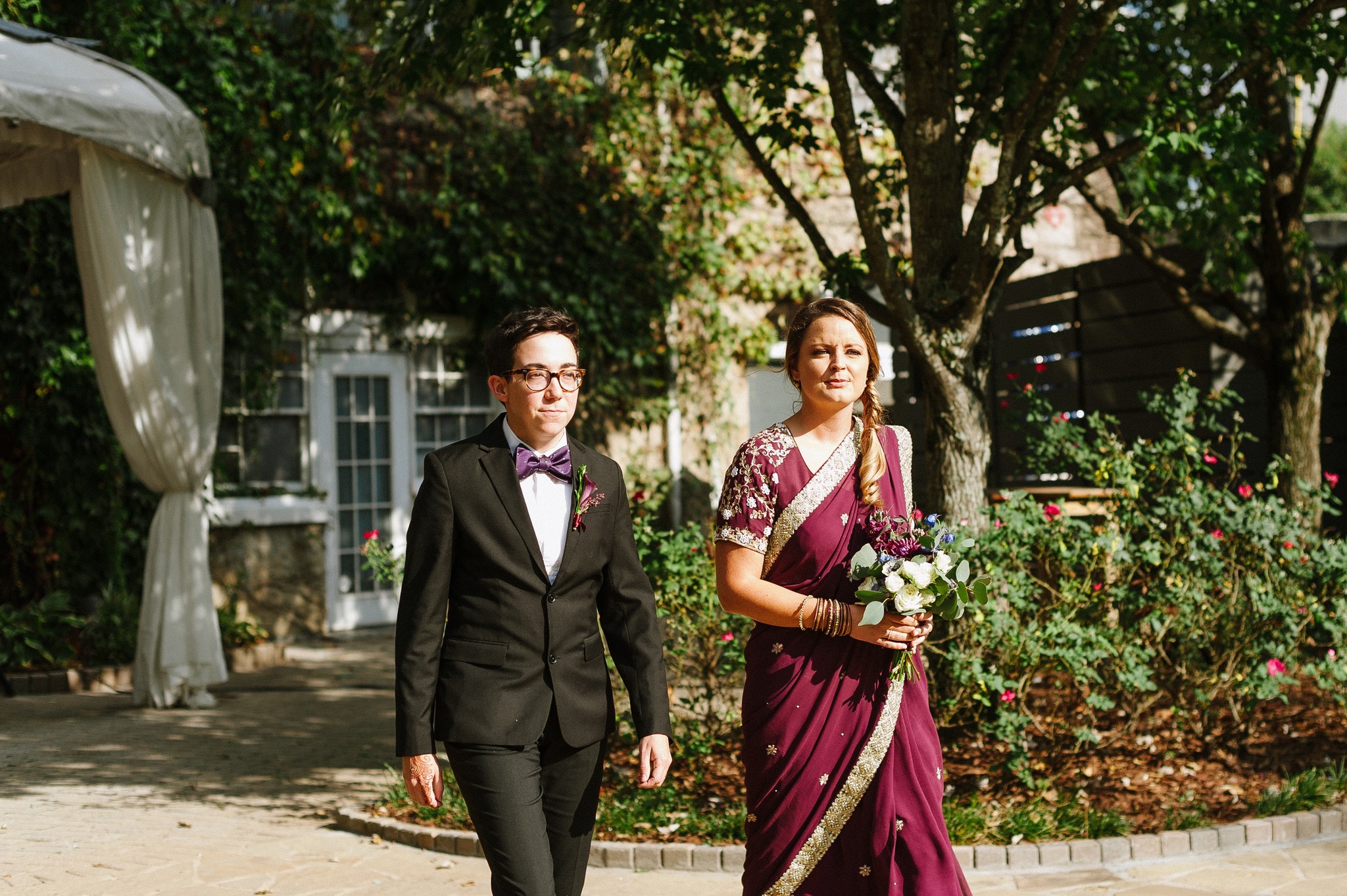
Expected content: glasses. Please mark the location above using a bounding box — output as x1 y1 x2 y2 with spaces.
497 368 587 392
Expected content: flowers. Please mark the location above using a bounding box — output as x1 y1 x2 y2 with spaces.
571 463 603 531
847 503 971 683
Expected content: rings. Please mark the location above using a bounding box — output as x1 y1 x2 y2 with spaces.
887 630 892 641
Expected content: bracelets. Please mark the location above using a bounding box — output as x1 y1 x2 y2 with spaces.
795 592 854 642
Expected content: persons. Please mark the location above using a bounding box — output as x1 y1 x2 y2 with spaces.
390 303 674 894
713 299 977 896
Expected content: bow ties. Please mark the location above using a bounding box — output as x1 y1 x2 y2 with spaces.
516 444 573 486
